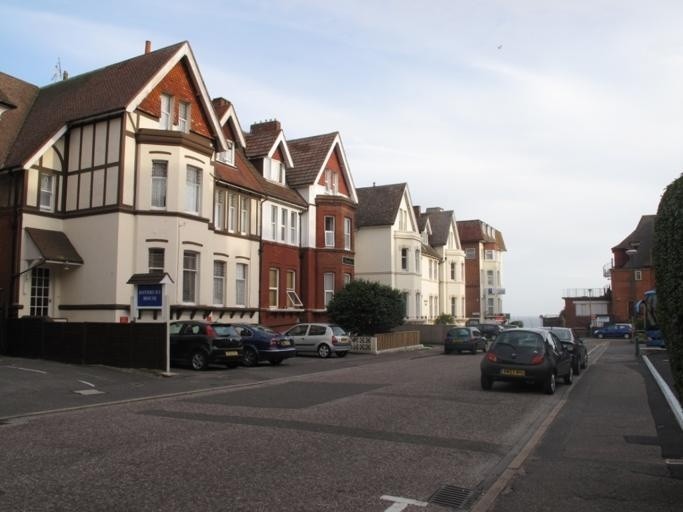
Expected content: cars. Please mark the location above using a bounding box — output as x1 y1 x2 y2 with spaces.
444 326 488 354
169 320 244 371
276 322 352 358
532 326 588 376
503 325 518 329
480 327 575 394
227 323 296 367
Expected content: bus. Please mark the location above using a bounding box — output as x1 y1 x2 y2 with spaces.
635 288 666 349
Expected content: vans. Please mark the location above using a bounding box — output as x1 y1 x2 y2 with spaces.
508 321 523 328
465 323 503 341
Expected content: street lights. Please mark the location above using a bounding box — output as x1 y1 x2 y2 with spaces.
625 249 640 357
588 288 592 337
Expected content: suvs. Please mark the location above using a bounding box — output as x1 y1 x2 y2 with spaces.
592 323 635 339
